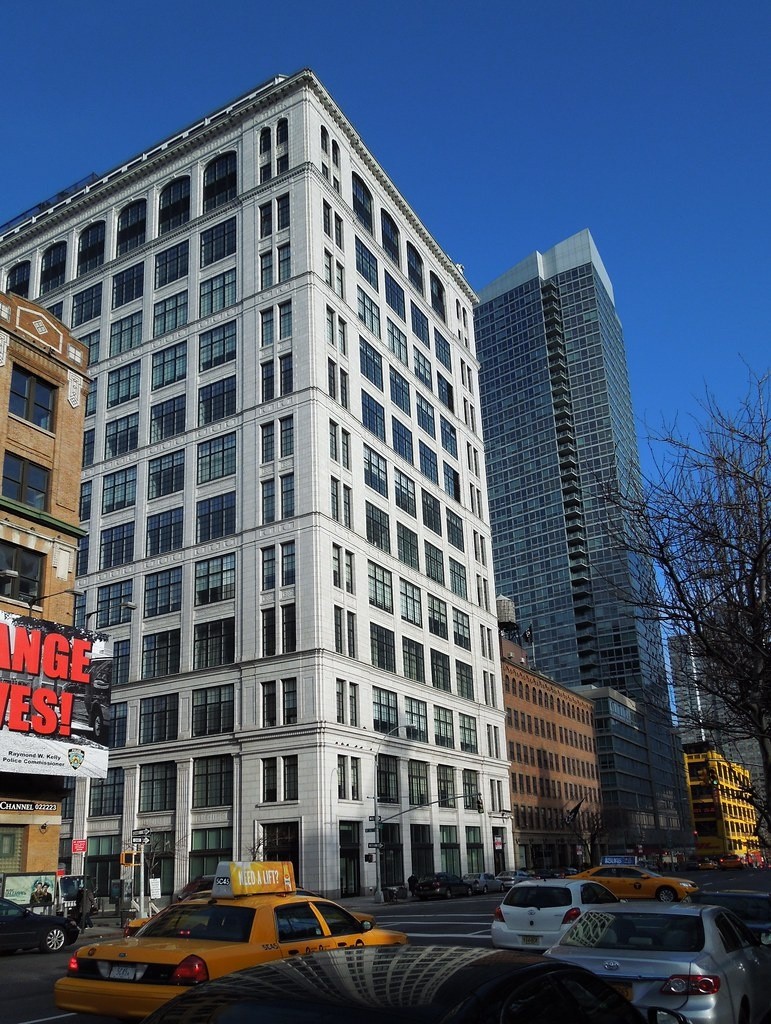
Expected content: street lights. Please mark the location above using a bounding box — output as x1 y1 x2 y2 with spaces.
374 724 417 904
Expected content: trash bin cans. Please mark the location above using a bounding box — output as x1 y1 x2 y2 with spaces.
382 886 394 901
397 886 408 899
121 909 137 929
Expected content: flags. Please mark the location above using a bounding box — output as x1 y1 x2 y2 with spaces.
565 797 584 825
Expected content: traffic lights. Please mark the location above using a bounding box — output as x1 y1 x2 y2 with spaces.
364 854 373 862
697 768 707 783
133 851 141 866
709 767 719 785
123 852 133 865
477 800 484 813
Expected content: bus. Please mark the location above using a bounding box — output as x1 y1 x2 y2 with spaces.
747 849 762 867
57 874 98 916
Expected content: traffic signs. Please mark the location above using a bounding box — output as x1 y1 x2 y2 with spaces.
131 836 151 844
132 827 151 837
369 816 382 821
368 842 385 849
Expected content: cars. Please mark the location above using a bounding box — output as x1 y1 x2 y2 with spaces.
495 871 534 888
174 873 217 903
138 944 695 1023
122 887 377 939
491 878 628 952
464 872 505 894
518 863 593 880
720 853 745 871
53 860 410 1020
565 855 700 903
674 860 699 871
540 900 771 1024
698 858 719 870
414 872 473 901
0 896 79 953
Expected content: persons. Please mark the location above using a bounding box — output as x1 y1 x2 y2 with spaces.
74 884 97 930
663 861 667 870
669 861 672 872
30 882 46 903
42 882 52 902
408 872 418 897
637 861 641 867
